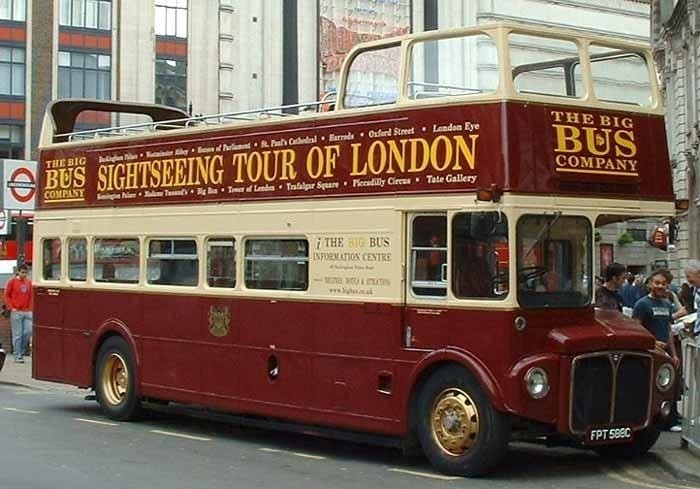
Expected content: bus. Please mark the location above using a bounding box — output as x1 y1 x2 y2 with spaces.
31 20 678 474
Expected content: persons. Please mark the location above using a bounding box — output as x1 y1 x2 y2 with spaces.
594 257 700 434
4 264 34 365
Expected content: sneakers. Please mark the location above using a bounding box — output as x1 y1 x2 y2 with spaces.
669 424 683 432
14 356 27 364
